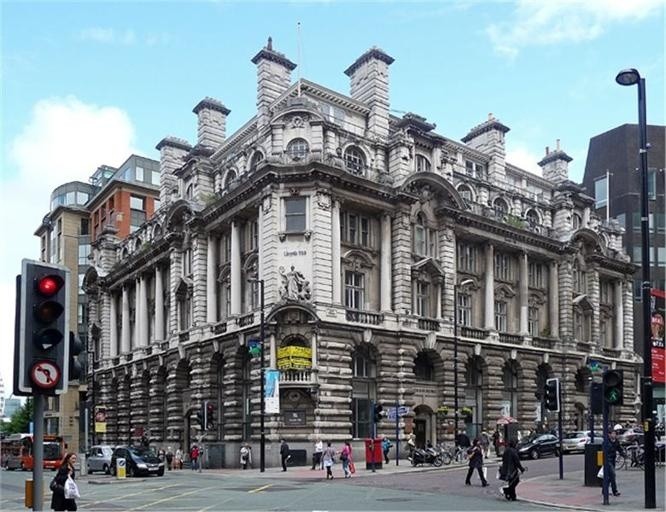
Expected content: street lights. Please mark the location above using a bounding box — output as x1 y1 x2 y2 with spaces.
453 278 474 462
613 68 660 509
246 277 266 473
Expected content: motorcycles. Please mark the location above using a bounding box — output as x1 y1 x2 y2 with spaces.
407 448 443 467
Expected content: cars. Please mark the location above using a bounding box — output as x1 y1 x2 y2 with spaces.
615 426 645 444
561 429 605 454
109 445 165 478
515 432 562 461
86 444 118 473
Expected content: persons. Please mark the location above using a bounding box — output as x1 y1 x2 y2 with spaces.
457 428 527 500
530 428 536 436
603 430 628 498
311 437 354 480
157 446 199 471
51 453 77 511
283 265 305 302
240 443 252 469
383 437 390 465
280 438 290 471
408 430 416 461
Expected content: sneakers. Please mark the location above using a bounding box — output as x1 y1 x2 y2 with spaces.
326 476 334 479
609 491 620 496
465 480 489 486
345 472 351 478
504 487 517 501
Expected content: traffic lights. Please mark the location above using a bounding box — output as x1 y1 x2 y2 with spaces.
372 404 383 424
206 402 215 429
603 371 623 404
66 331 85 380
32 273 67 352
544 382 556 411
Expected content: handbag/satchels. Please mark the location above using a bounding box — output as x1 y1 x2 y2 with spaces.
340 453 347 460
49 479 64 492
243 455 248 460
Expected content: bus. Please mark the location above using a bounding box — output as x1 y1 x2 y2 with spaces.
0 432 66 471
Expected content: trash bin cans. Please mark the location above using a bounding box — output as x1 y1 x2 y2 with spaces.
584 444 607 487
116 458 127 479
25 479 45 508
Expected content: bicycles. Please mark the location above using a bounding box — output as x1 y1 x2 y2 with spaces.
612 437 658 470
437 446 467 466
